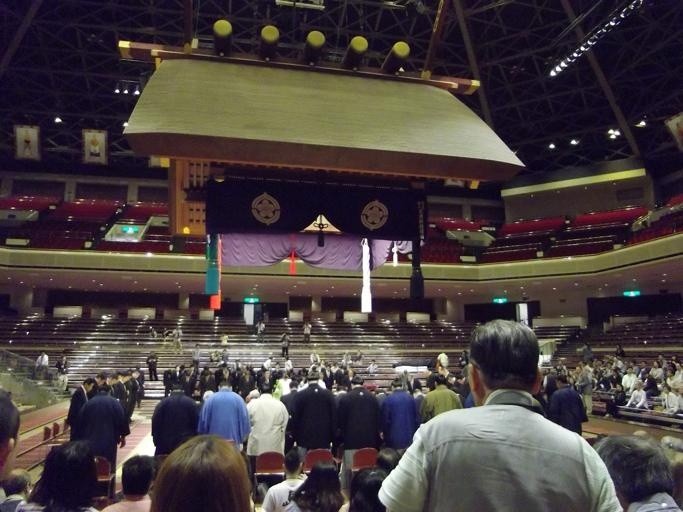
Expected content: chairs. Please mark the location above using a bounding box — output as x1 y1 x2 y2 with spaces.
302 447 333 474
351 444 378 474
38 424 62 470
254 447 285 494
91 452 116 496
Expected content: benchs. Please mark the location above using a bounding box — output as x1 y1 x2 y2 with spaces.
0 192 205 255
591 312 683 347
532 325 590 371
417 194 683 264
5 318 472 347
2 348 468 399
593 355 683 426
